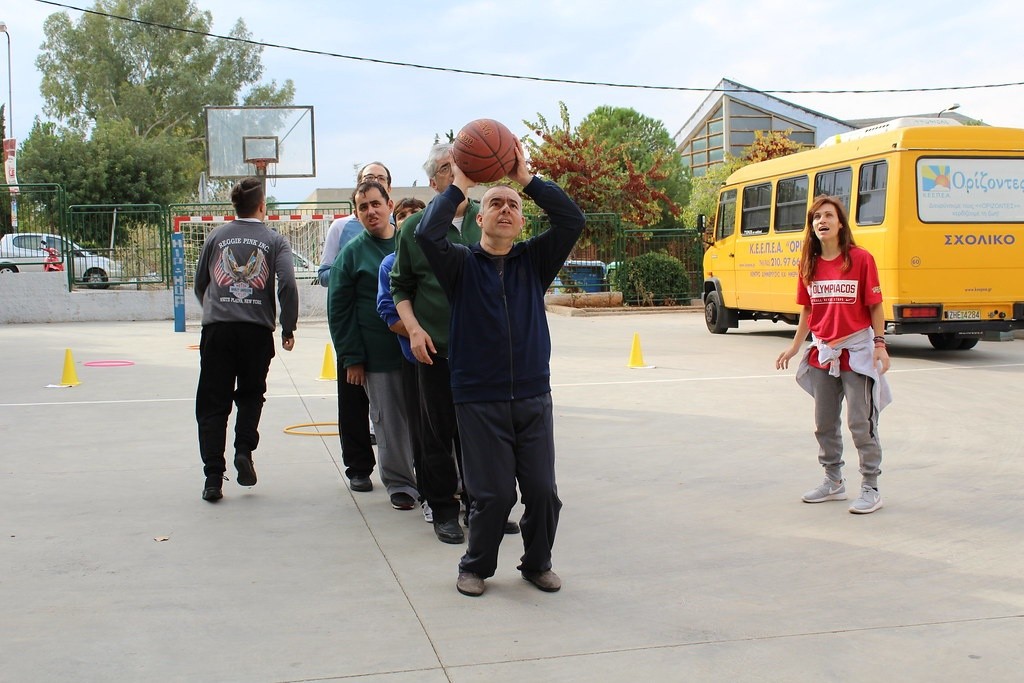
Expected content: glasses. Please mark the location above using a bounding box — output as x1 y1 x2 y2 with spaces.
432 164 450 178
363 174 388 184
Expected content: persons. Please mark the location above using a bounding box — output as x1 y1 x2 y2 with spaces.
776 196 892 513
193 176 299 502
388 143 520 543
325 180 465 511
376 198 467 522
316 162 418 491
413 136 586 595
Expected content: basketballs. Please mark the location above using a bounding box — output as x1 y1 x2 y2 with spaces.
452 118 517 183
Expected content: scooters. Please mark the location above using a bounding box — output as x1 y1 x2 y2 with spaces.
38 239 64 271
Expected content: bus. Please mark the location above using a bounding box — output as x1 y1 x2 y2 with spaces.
697 118 1024 351
553 260 624 295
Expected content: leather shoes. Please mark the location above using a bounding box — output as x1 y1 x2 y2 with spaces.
434 519 465 544
504 520 520 534
350 475 373 491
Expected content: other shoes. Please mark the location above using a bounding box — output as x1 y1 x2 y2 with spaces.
202 487 223 499
234 451 256 486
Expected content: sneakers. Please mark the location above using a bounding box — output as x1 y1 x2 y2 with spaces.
802 477 846 503
849 485 883 513
456 571 485 596
391 492 414 509
522 569 561 591
418 500 433 522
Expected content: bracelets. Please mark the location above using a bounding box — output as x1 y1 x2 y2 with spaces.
873 336 886 348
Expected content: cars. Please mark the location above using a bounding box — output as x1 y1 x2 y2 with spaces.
0 233 123 289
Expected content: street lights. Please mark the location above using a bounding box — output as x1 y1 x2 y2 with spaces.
0 20 18 234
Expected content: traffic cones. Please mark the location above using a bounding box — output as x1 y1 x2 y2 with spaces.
318 343 338 380
57 347 82 388
626 331 648 369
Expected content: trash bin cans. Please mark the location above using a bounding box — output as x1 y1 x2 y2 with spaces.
607 262 623 292
555 261 606 295
